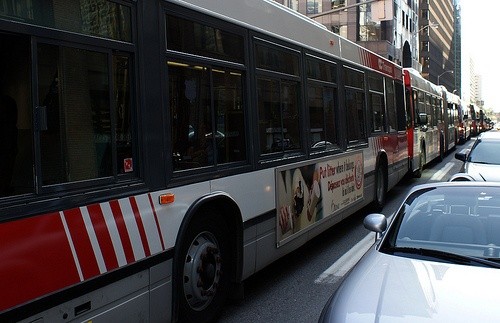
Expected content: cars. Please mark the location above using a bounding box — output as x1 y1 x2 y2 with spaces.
455 131 500 182
318 173 500 323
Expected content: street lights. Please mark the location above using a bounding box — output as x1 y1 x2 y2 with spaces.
437 70 454 85
410 24 440 68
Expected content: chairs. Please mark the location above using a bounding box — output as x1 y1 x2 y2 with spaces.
429 214 486 245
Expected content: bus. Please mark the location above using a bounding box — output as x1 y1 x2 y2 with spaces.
0 0 493 323
